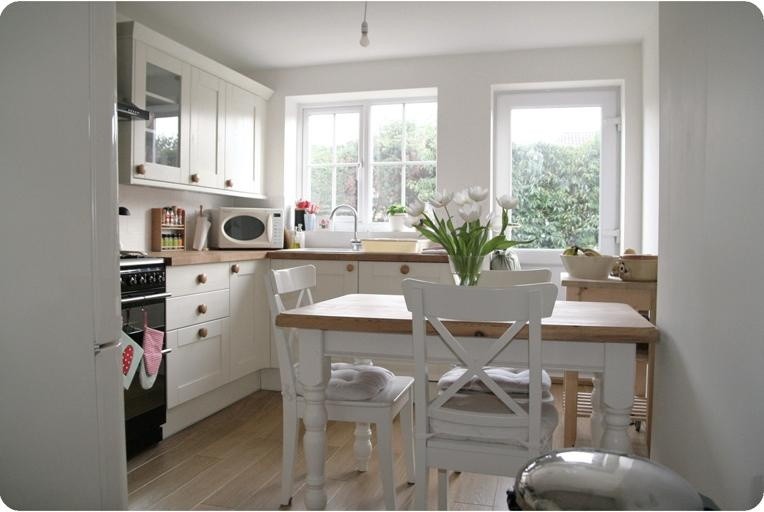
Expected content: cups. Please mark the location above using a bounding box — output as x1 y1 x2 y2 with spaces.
284 224 296 249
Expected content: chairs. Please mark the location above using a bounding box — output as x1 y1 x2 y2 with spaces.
400 277 559 510
265 264 416 511
435 267 555 476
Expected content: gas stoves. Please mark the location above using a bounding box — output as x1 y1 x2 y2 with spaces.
120 250 165 290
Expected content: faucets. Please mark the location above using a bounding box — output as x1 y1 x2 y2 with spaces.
329 204 359 240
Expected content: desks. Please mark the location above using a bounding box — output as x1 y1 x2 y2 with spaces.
559 269 658 460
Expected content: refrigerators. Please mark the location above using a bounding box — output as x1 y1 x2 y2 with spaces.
0 0 131 512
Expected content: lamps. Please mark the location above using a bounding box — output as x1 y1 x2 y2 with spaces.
358 2 370 48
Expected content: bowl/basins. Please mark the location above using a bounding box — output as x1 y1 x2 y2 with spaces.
558 252 619 280
620 253 658 282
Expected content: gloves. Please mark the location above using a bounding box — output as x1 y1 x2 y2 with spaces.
139 326 165 390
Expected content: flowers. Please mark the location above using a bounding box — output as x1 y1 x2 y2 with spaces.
402 184 537 285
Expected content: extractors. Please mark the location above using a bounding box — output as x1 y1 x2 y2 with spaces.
111 95 151 122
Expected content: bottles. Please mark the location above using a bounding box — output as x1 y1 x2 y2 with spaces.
161 206 182 225
160 233 183 250
296 224 305 247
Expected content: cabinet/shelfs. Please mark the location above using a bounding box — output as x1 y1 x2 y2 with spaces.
166 264 230 439
230 257 265 408
117 40 191 191
191 65 264 200
266 259 449 423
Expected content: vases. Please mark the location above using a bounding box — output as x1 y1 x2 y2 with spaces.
445 255 486 287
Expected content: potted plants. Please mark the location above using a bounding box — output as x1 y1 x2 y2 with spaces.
387 205 409 231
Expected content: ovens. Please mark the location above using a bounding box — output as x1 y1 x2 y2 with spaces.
117 289 171 426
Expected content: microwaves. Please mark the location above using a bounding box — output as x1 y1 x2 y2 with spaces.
205 206 284 249
